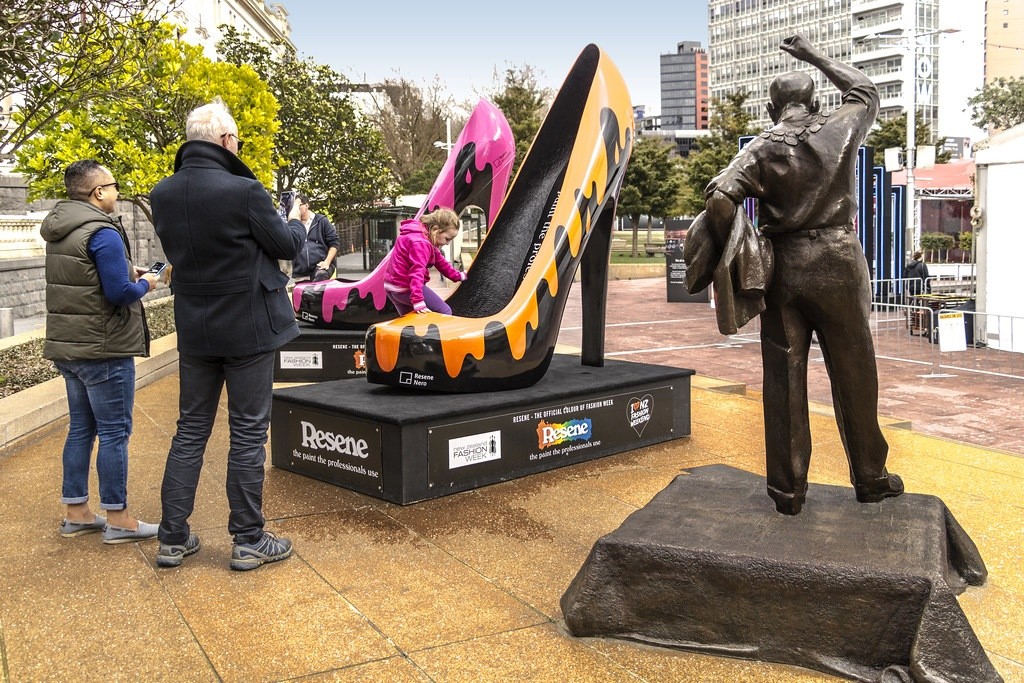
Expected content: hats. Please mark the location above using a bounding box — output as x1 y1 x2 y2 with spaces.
914 252 922 260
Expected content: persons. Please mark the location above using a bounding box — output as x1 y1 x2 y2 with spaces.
904 252 931 311
150 105 306 571
384 208 467 317
706 34 905 515
290 194 339 282
40 160 162 546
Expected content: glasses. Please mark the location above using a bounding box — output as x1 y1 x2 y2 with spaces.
220 133 245 150
88 182 119 196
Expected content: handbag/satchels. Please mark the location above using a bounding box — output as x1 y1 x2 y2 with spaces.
923 285 931 294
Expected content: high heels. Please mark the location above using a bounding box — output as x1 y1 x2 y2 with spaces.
290 94 515 327
366 42 635 390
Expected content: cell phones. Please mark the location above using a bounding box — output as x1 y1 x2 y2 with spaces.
147 261 167 277
279 191 296 221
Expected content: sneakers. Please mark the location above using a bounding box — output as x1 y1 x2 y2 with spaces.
156 533 201 566
103 519 160 544
61 514 107 538
230 532 292 572
